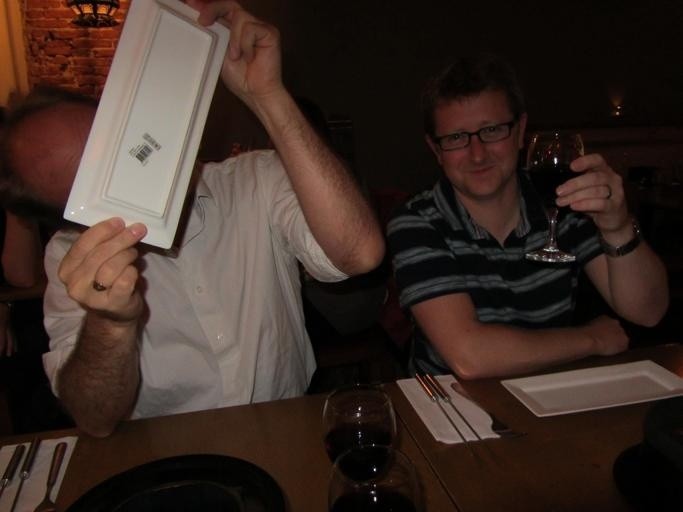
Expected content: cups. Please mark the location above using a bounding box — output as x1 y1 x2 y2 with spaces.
322 387 418 511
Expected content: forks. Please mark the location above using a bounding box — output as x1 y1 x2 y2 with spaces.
450 381 528 438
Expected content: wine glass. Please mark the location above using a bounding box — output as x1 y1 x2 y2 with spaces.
524 129 586 263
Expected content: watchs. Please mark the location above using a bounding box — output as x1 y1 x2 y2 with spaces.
595 214 643 257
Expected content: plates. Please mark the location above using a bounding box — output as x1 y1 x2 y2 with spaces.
500 359 683 418
60 453 292 512
62 0 231 252
612 436 683 512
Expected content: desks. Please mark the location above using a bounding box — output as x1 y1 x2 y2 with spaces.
0 343 683 512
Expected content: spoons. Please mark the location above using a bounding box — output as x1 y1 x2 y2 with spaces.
33 442 66 512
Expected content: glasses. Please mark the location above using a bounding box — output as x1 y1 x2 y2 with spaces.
434 119 517 151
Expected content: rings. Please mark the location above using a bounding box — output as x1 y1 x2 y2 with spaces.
604 184 612 199
92 279 112 293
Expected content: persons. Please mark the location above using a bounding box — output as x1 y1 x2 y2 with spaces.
1 204 43 436
386 60 669 384
0 0 385 437
